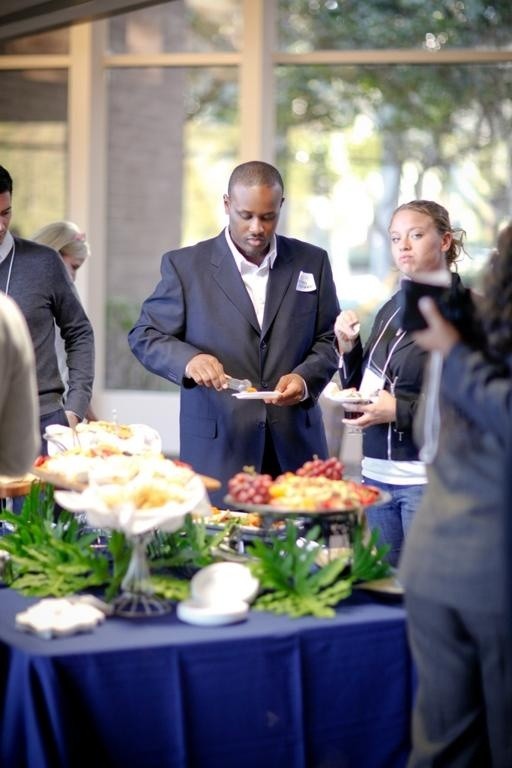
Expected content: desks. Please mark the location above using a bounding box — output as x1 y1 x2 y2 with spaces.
0 564 409 767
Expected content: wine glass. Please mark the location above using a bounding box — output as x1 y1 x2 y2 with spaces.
342 401 368 436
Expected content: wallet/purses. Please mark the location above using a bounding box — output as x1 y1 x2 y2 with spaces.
400 278 456 332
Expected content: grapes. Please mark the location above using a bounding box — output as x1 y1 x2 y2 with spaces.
296 454 343 481
229 472 273 505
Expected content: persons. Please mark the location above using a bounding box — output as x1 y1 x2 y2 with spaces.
1 160 99 465
390 222 510 767
404 247 510 452
26 221 90 289
127 159 345 510
330 197 492 577
0 286 44 479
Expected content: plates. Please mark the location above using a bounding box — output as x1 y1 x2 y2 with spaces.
231 391 282 400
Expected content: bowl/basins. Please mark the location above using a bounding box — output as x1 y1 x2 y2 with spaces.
190 560 260 609
174 593 251 629
